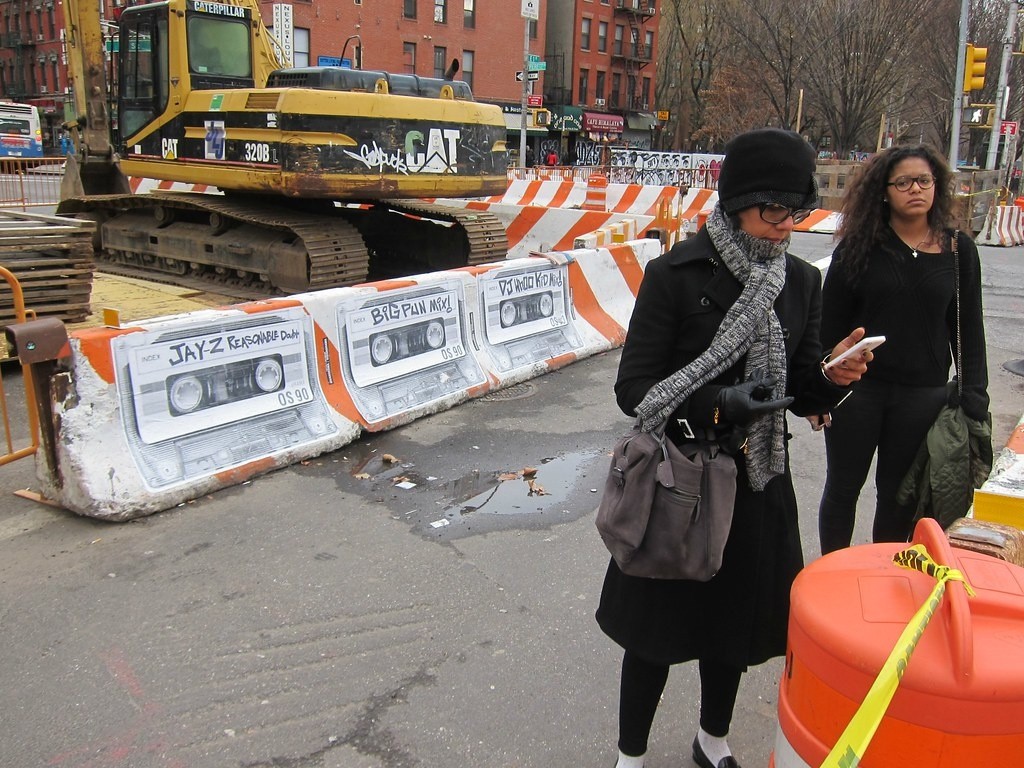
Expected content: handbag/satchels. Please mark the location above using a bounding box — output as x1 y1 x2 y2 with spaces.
595 415 737 582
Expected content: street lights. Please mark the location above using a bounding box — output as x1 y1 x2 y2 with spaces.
650 125 656 150
662 126 667 152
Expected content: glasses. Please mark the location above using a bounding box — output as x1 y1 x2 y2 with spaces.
887 175 937 192
757 203 811 225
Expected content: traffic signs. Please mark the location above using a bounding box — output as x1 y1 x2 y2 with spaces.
515 71 539 82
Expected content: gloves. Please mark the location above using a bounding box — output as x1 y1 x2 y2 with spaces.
717 372 796 429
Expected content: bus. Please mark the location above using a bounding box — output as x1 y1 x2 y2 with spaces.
0 102 42 171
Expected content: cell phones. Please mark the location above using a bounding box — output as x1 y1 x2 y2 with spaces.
821 334 888 370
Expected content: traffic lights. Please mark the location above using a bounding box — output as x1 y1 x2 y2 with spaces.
963 47 987 91
962 106 983 125
532 110 551 127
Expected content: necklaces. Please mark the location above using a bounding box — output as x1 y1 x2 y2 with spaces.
888 219 931 257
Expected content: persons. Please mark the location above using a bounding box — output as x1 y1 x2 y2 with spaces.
806 143 990 555
595 128 874 768
525 145 558 168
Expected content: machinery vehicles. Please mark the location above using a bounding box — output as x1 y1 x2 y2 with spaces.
55 1 508 298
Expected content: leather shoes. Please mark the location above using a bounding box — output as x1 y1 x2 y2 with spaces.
613 755 646 768
692 733 742 768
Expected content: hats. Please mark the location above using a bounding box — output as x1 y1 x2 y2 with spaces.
718 128 819 215
